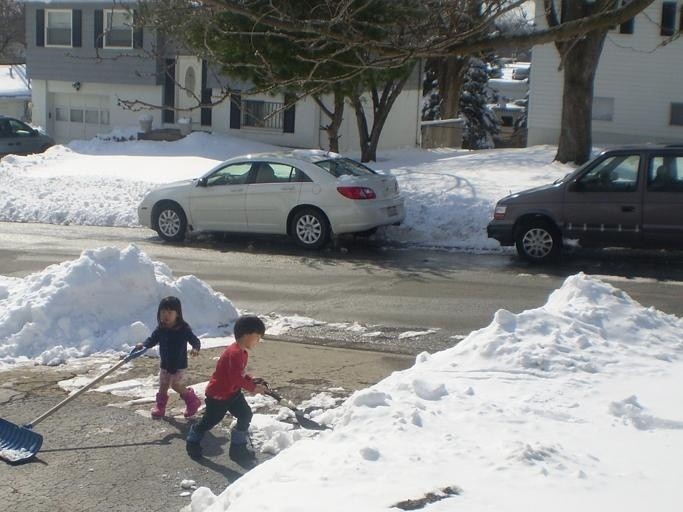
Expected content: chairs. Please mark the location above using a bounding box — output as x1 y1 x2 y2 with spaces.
651 163 668 189
257 164 276 182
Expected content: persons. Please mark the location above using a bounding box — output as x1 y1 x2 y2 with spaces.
184 313 270 459
134 294 201 417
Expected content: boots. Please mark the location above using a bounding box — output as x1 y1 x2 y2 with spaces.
186 419 205 447
229 426 255 459
151 393 168 419
180 387 201 417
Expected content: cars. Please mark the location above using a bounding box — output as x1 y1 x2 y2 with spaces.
0 112 55 158
135 148 408 253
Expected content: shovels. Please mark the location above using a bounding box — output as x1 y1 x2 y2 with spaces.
254 382 327 431
0 346 148 463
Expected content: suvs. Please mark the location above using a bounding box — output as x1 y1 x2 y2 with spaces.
485 146 683 267
